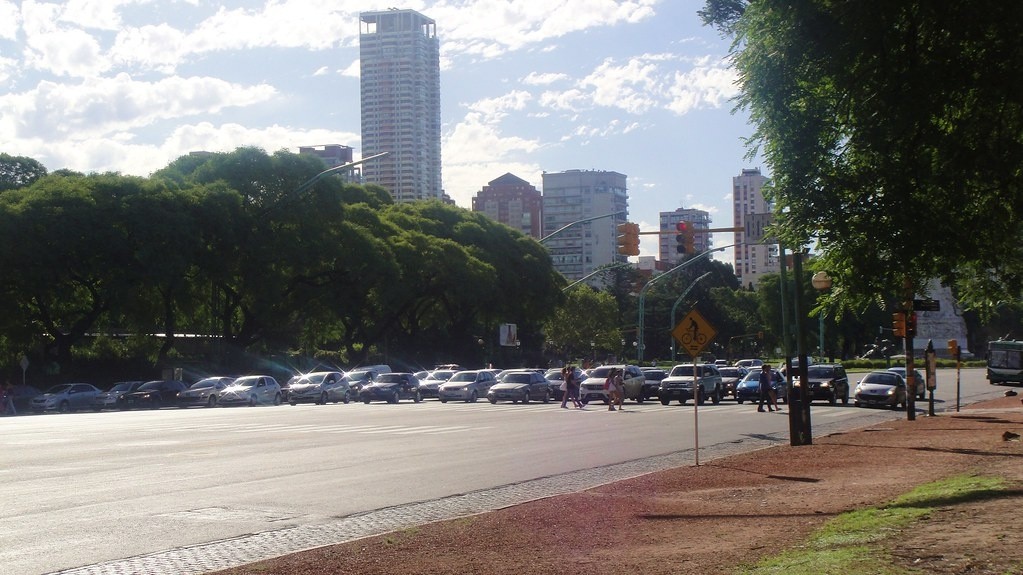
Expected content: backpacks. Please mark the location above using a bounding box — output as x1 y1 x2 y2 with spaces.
605 378 612 389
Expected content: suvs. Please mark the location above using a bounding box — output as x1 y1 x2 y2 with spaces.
579 363 645 404
658 361 723 405
792 362 849 406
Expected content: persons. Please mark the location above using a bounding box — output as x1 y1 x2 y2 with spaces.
607 368 625 411
0 381 17 415
561 366 586 409
757 364 782 412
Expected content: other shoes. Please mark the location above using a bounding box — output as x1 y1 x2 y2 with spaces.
776 408 781 411
758 408 765 412
561 404 567 408
574 404 579 407
579 404 586 408
769 409 775 412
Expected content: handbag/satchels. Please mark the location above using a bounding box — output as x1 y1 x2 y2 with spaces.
560 381 567 391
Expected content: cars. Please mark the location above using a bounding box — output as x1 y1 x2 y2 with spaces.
95 379 187 411
29 383 105 414
280 363 584 406
175 375 283 408
714 356 813 404
854 367 926 409
639 366 669 400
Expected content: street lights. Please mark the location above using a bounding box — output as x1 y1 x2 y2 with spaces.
812 271 833 363
671 271 713 365
561 262 634 292
538 210 626 244
638 247 726 363
621 339 626 363
257 150 390 216
591 341 595 366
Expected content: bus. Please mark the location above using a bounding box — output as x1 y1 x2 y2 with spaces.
987 329 1023 385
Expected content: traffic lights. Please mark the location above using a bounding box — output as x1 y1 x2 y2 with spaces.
892 313 906 337
759 331 763 339
905 312 917 338
616 222 633 255
947 339 957 355
676 218 694 254
